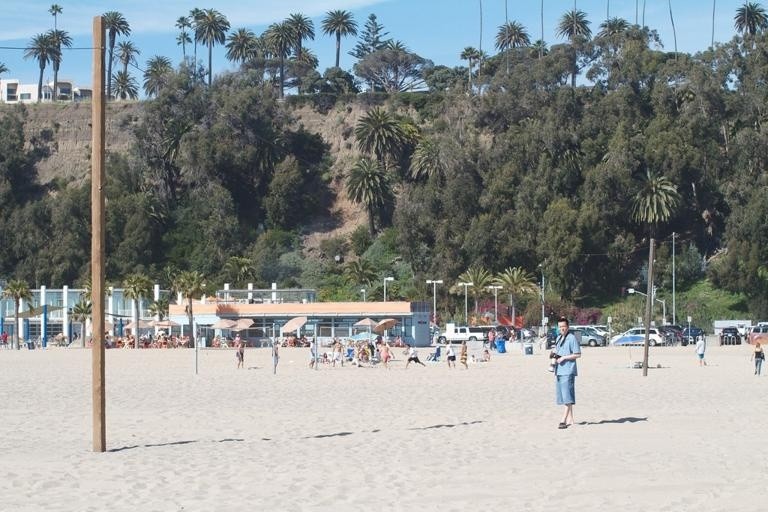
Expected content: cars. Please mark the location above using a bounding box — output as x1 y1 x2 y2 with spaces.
745 322 768 345
546 324 706 347
721 327 741 344
434 326 535 344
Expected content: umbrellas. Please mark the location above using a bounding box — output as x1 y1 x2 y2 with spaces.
209 318 237 329
230 318 255 333
147 319 177 326
281 316 308 333
122 319 153 329
353 317 378 326
373 318 399 333
105 320 113 333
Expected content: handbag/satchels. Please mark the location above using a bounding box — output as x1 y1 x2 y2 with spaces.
761 353 764 359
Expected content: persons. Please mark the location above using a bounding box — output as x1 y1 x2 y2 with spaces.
509 326 516 342
482 349 490 361
236 342 245 368
750 342 765 376
212 335 221 347
458 340 469 368
488 328 497 351
551 318 581 429
0 331 81 349
401 344 426 368
271 334 403 374
90 331 190 349
232 333 241 348
445 344 457 368
694 335 707 366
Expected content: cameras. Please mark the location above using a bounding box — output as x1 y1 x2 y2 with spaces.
548 353 561 372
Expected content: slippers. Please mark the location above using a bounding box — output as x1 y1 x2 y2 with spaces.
559 423 567 428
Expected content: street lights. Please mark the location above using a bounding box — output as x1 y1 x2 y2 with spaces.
457 282 473 323
488 285 503 321
539 263 549 328
360 289 366 302
425 279 443 325
625 288 666 325
383 276 395 302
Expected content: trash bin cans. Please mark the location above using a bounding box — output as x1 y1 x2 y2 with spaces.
525 343 533 354
681 337 687 346
28 341 34 350
197 335 206 347
496 339 505 353
